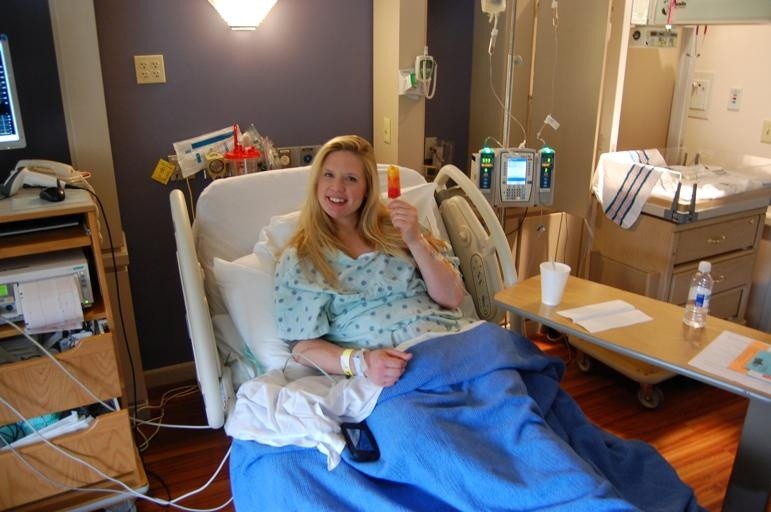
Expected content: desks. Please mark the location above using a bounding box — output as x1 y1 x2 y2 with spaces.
492 270 771 406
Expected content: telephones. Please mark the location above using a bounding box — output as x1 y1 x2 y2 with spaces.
13 156 82 186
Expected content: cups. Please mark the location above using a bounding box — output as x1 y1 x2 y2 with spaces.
223 143 258 177
539 262 570 306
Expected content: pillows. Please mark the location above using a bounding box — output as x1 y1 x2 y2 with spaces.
213 181 436 380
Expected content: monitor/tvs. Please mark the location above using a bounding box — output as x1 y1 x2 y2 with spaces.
0 32 27 148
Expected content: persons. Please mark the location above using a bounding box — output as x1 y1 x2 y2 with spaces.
268 135 477 386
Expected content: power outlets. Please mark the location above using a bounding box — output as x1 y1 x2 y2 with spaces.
135 54 165 88
760 120 771 143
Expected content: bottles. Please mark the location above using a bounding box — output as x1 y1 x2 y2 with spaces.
682 261 714 327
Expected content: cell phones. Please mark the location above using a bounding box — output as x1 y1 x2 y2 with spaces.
341 422 382 461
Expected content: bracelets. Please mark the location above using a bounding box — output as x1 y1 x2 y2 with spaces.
353 349 367 380
339 347 355 378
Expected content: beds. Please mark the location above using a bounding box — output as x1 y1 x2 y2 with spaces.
166 161 710 512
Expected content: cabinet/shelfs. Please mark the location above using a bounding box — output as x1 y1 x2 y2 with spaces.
582 182 770 409
1 182 152 511
476 210 585 280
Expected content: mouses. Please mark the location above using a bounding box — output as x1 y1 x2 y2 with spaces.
40 187 66 202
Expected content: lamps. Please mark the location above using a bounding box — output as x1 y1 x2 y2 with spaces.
209 1 278 32
